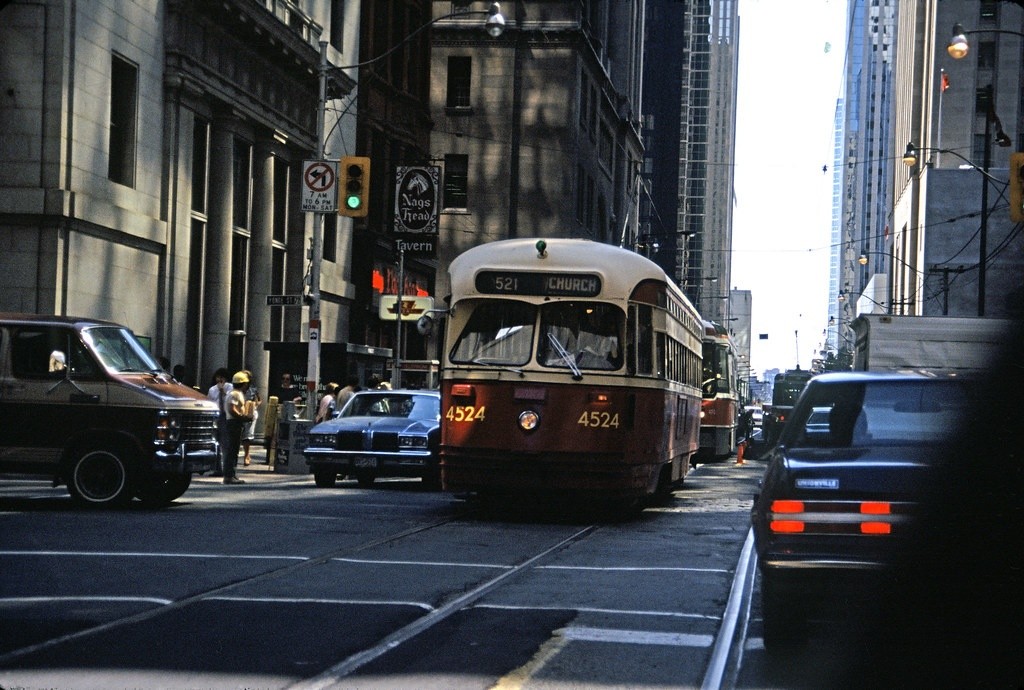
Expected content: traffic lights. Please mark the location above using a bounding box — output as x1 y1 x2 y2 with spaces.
337 156 372 219
1008 151 1024 224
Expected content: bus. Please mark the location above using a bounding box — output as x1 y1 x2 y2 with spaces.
770 330 821 406
692 320 746 460
414 172 705 521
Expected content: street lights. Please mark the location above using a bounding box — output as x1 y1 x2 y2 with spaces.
306 1 508 421
858 249 951 317
902 84 995 317
836 288 894 315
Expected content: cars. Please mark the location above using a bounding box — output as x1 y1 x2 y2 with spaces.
748 365 993 659
304 391 443 490
744 405 833 444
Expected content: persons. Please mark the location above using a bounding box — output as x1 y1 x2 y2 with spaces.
223 374 254 485
276 374 301 403
735 409 756 465
314 380 420 422
162 359 261 476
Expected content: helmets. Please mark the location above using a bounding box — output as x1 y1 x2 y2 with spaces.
232 371 249 384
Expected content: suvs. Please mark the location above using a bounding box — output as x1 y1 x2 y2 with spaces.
0 312 222 514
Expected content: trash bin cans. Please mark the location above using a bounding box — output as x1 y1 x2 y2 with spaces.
274 416 313 476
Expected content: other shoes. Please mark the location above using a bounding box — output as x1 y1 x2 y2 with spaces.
244 456 251 466
209 472 224 477
224 476 245 484
